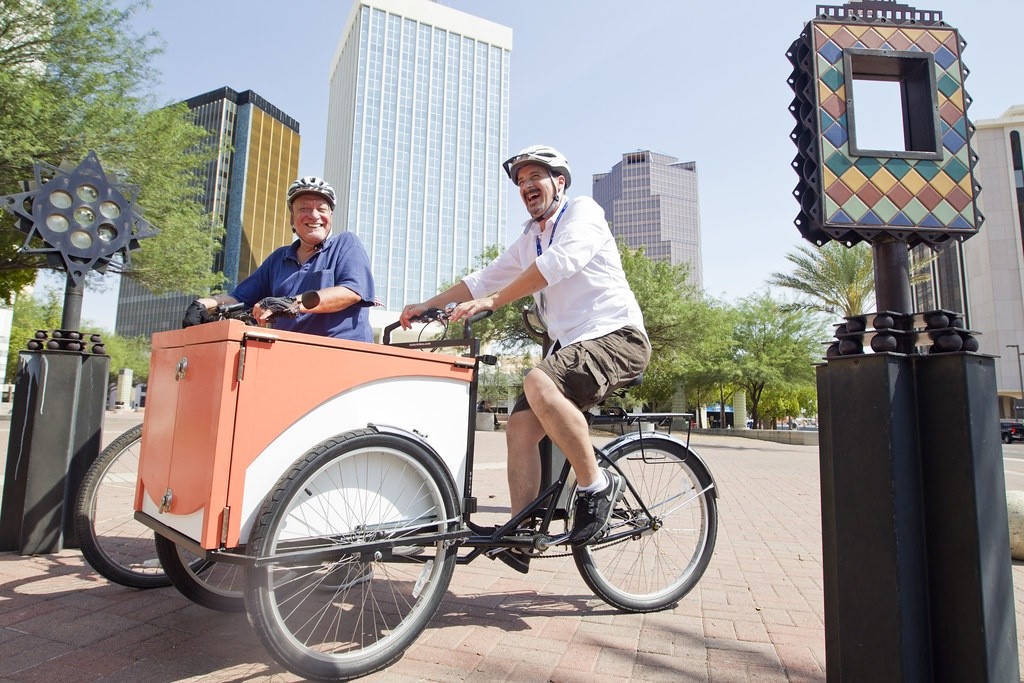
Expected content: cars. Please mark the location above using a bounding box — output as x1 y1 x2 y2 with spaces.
1001 422 1024 443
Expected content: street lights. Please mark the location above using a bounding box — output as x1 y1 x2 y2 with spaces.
1007 344 1024 406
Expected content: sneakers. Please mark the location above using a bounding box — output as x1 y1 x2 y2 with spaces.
468 522 534 573
570 467 626 548
322 558 373 589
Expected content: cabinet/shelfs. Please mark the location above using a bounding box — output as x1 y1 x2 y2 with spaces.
132 317 478 553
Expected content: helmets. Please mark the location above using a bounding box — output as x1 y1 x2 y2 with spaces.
502 145 573 191
286 176 336 210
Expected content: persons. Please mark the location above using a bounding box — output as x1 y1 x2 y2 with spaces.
401 145 652 573
478 401 500 426
182 177 375 343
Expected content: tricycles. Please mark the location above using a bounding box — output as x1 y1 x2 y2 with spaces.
69 289 720 681
